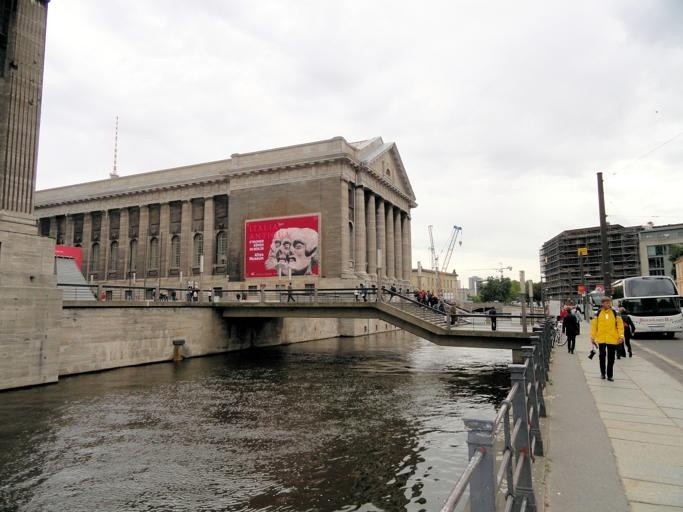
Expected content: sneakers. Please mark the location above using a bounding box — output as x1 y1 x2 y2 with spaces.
607 377 613 381
601 375 605 379
568 347 574 354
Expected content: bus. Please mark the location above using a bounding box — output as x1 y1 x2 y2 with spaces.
586 287 604 320
609 274 683 341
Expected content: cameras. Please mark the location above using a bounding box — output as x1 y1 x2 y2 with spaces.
588 347 597 360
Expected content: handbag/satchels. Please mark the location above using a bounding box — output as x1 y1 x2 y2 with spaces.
623 322 628 328
576 321 580 335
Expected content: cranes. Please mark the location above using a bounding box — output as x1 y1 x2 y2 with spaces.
484 261 513 280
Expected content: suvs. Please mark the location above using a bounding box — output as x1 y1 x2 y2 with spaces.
574 296 584 314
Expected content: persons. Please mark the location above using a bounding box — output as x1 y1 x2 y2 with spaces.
287 282 295 303
560 305 583 354
591 296 636 381
208 287 212 302
152 289 176 301
185 287 199 302
414 289 457 324
353 283 396 303
100 290 107 301
488 307 497 331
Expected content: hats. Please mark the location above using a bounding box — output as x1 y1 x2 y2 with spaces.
564 306 572 310
